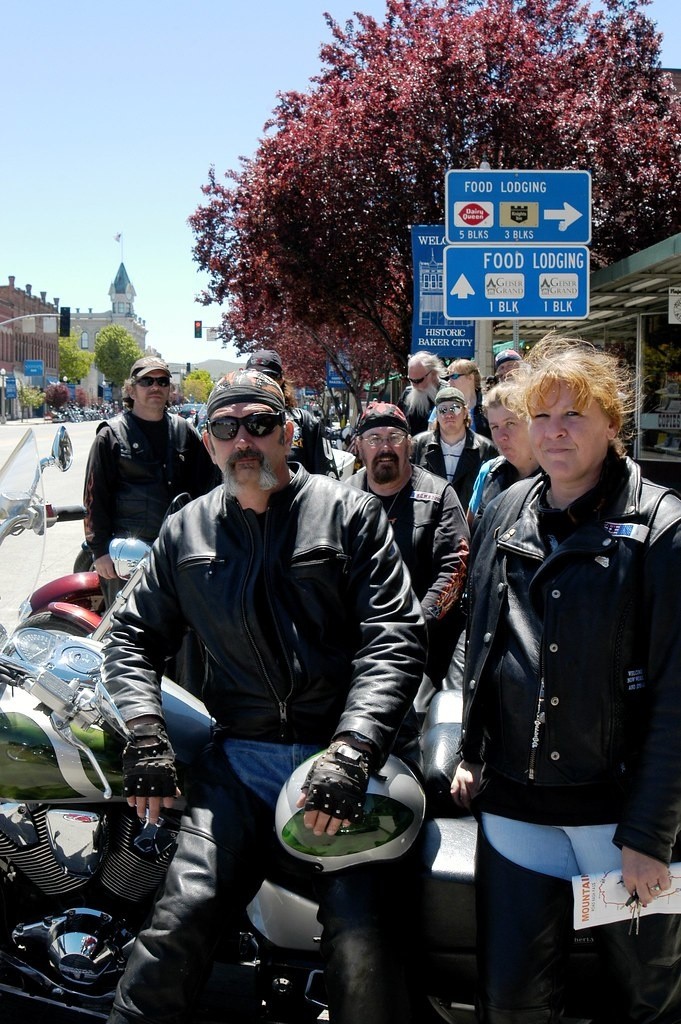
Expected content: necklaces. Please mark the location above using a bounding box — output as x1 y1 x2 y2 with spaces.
367 473 404 515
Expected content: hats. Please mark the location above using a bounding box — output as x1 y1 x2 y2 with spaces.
355 401 409 436
495 350 521 371
435 387 465 404
207 370 285 422
130 356 172 386
246 350 283 378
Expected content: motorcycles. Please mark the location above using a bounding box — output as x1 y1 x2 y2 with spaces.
0 424 480 1024
50 404 114 422
167 405 182 413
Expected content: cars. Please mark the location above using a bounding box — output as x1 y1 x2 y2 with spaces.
178 404 209 430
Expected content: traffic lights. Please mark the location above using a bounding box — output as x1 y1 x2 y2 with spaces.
194 321 202 338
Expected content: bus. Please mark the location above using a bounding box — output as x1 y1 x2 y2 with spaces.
365 338 528 404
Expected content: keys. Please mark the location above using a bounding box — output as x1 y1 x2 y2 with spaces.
621 890 641 935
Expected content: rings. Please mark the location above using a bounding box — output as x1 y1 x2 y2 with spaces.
651 884 659 891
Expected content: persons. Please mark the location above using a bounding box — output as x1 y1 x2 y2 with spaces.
245 349 545 816
451 332 681 1024
83 357 222 611
101 369 427 1024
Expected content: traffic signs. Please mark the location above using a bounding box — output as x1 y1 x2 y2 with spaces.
442 171 593 322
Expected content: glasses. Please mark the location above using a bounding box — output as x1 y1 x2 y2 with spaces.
438 404 460 413
367 432 404 446
444 373 464 381
138 376 170 387
410 371 431 384
208 412 280 441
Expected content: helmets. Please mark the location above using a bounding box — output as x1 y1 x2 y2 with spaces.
273 747 425 873
